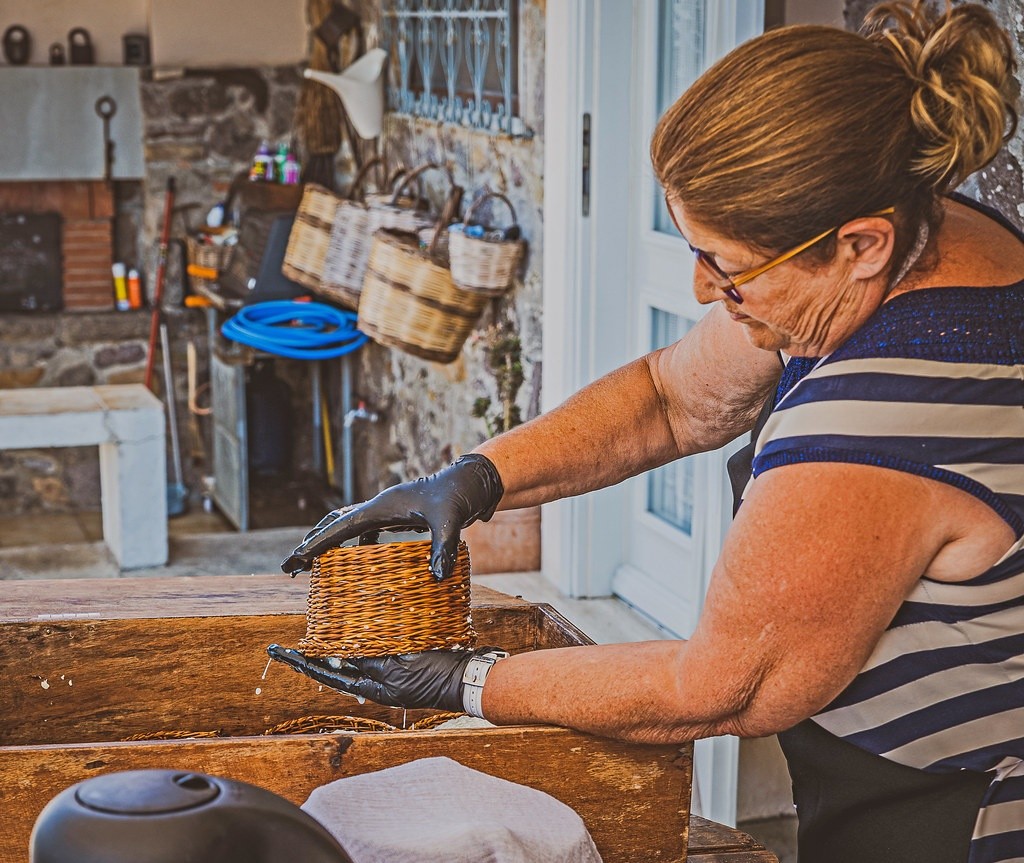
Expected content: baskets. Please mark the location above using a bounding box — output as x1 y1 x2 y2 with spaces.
264 715 399 733
281 158 524 365
297 541 476 660
407 709 470 730
122 730 225 740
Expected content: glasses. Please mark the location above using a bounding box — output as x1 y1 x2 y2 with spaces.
666 197 896 305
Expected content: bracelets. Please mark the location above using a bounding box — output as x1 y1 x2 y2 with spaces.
463 651 510 719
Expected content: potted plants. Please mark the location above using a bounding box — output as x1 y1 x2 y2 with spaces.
455 330 539 575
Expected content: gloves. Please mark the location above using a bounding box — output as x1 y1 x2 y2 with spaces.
280 450 504 583
267 639 495 714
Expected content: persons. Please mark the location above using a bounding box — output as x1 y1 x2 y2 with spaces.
270 0 1024 863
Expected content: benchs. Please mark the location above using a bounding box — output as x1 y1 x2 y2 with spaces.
0 383 170 571
